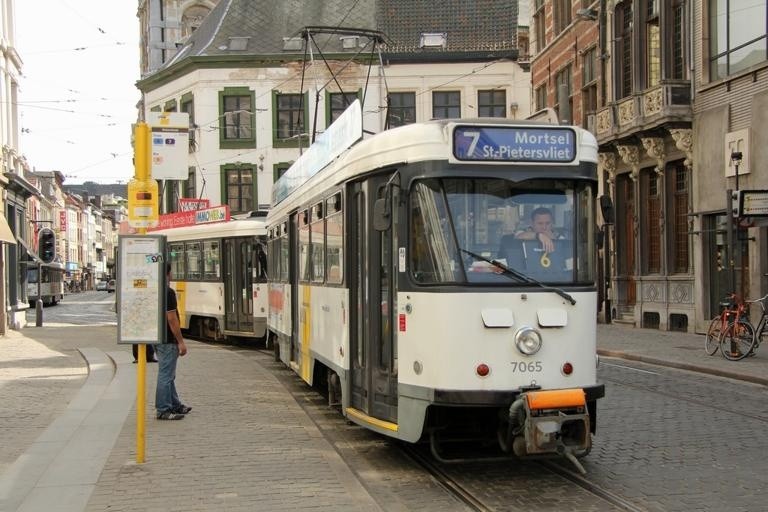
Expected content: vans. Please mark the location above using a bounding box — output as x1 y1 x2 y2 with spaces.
108 279 116 293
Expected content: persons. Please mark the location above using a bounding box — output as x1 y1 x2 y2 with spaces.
63 270 92 292
131 343 158 363
155 264 193 421
512 205 566 252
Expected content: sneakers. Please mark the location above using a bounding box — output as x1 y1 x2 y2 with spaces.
131 358 159 363
156 410 185 421
172 404 192 414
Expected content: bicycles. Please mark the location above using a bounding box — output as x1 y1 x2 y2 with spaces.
705 292 768 361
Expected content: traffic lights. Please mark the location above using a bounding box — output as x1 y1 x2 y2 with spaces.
38 227 56 264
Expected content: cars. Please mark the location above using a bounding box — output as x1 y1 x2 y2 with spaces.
96 281 107 291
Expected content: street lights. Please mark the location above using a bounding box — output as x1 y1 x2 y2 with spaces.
576 9 611 324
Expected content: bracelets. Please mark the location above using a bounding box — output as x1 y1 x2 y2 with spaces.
534 231 541 240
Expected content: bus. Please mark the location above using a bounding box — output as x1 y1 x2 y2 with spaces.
147 107 605 476
28 263 66 308
